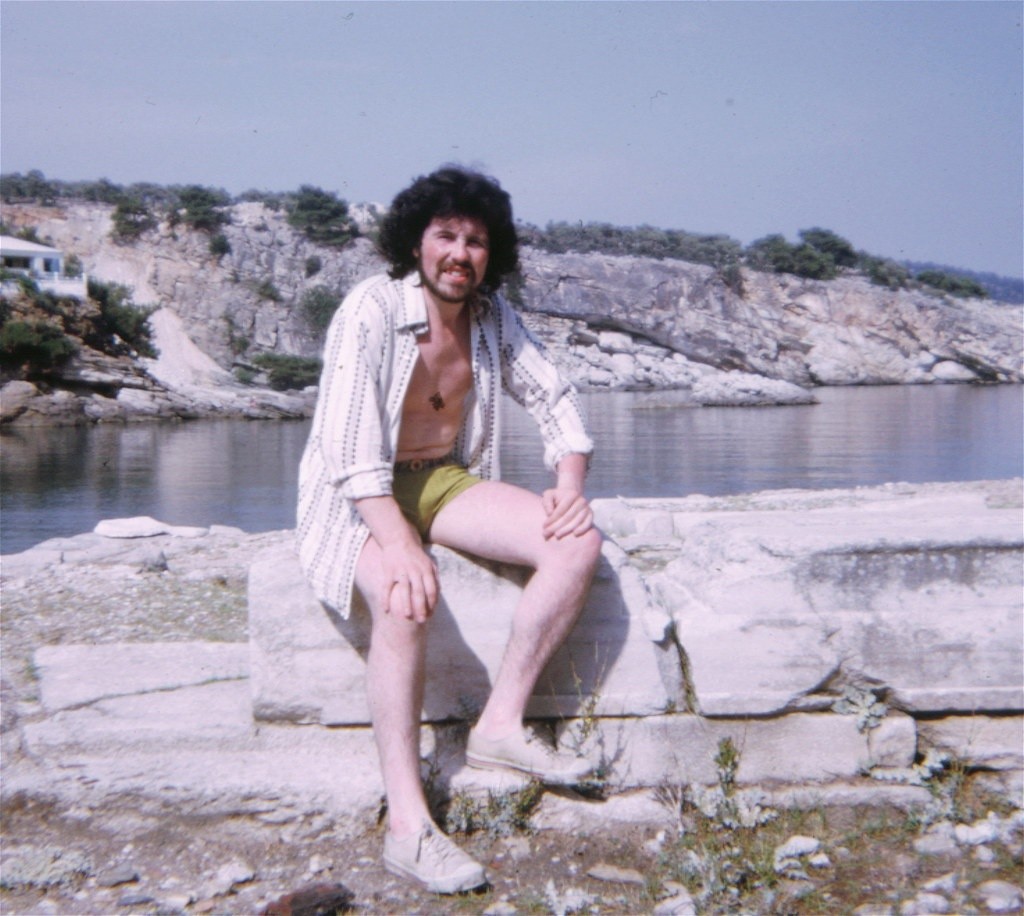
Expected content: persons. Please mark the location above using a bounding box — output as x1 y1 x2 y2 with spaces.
296 167 604 893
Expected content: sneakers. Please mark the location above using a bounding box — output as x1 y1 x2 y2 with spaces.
382 822 486 894
465 725 594 784
